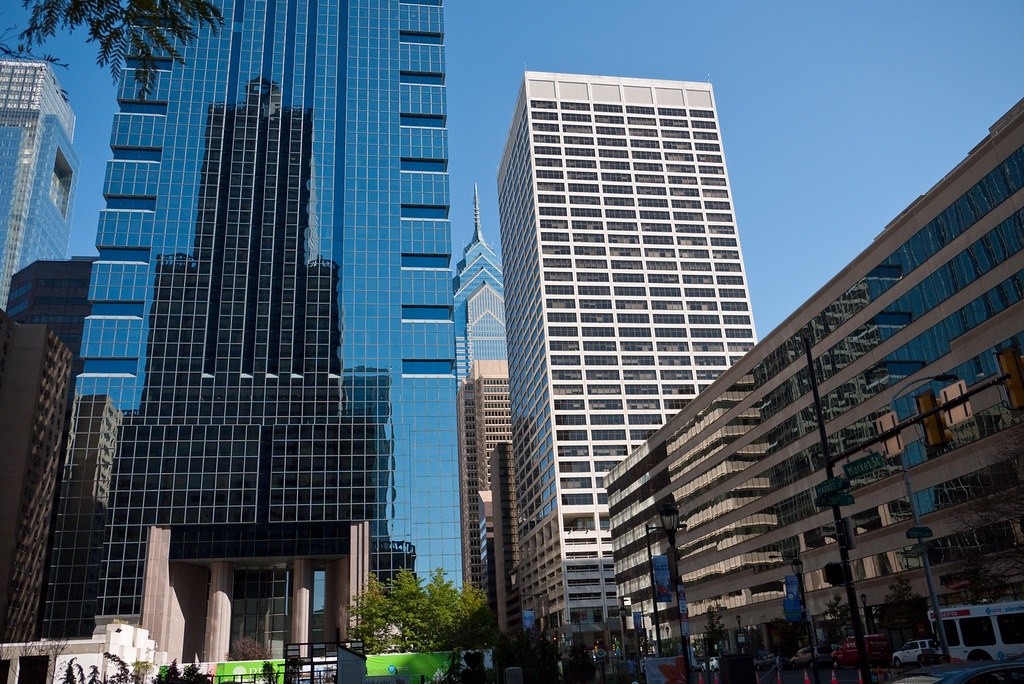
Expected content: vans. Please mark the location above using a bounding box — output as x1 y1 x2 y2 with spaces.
830 634 892 669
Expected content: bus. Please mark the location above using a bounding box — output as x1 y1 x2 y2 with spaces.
927 600 1024 669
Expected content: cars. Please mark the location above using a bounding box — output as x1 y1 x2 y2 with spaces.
891 639 938 668
692 657 719 673
790 645 831 669
753 653 779 671
880 657 1024 684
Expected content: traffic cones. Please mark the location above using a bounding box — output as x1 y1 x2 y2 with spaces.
832 670 839 684
804 670 810 684
715 672 719 684
777 670 782 684
858 669 864 684
698 670 704 684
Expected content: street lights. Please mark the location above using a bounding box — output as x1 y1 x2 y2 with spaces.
618 606 629 661
659 501 693 684
790 557 821 684
736 615 742 634
859 594 870 635
665 626 672 657
